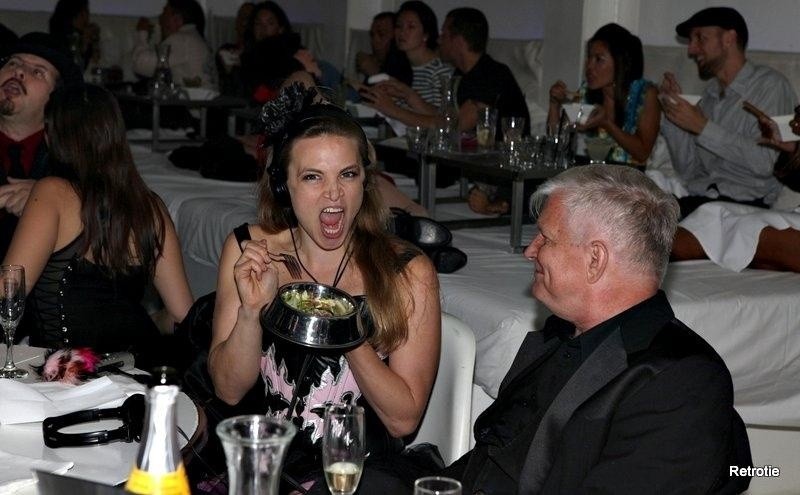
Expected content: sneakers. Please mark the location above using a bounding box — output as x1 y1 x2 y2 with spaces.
389 207 468 273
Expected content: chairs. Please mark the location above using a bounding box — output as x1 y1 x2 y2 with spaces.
413 308 476 464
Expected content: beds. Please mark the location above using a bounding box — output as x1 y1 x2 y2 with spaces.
113 86 797 430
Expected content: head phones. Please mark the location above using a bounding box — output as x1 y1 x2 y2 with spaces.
266 102 374 206
42 394 143 449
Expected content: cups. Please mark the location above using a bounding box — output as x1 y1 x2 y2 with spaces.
416 475 462 495
215 414 296 495
321 404 363 493
404 109 584 170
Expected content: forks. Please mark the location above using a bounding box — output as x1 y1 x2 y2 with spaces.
266 251 303 280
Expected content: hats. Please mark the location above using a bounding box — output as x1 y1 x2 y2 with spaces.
4 32 82 83
675 7 748 43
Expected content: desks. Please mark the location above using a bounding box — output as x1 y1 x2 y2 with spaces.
2 333 410 492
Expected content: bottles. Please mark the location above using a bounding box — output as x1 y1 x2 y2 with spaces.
148 40 175 100
434 75 466 151
124 366 195 495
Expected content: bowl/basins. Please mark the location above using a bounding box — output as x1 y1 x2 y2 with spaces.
661 94 702 108
261 282 367 350
218 48 242 68
561 102 598 126
770 115 799 142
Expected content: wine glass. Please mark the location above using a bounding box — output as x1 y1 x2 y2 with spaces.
1 265 31 384
